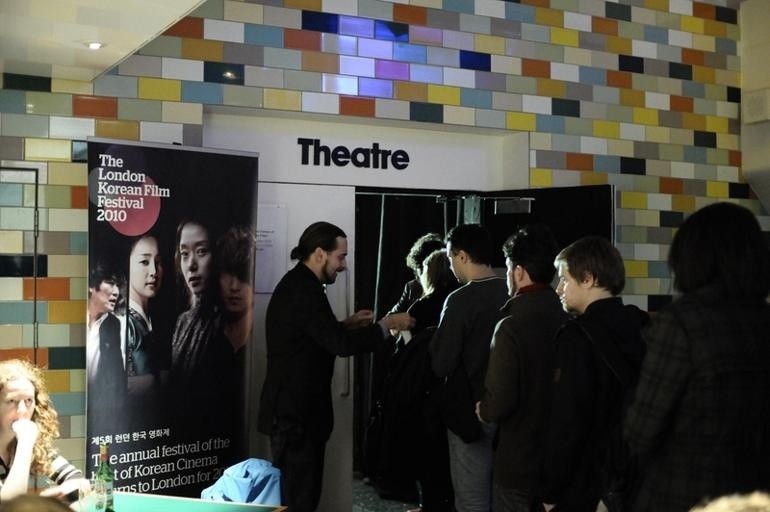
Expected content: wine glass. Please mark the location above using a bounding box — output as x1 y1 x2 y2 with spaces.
79 479 107 512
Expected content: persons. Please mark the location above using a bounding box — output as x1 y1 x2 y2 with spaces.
213 224 255 357
381 200 770 510
256 220 414 512
0 356 91 501
169 214 223 372
128 232 172 376
88 263 125 435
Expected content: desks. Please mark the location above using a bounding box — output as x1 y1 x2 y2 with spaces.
69 490 290 512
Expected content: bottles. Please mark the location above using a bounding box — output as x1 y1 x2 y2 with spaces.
95 442 114 512
